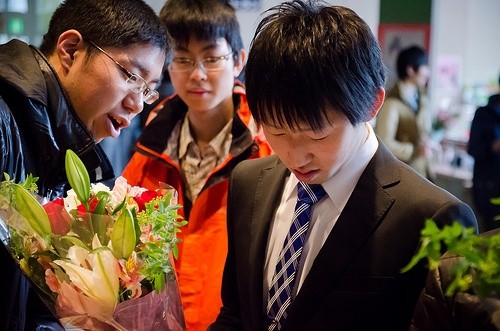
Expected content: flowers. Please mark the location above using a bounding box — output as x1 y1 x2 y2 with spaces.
0 148 189 331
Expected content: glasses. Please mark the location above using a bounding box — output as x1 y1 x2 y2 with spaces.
81 36 159 105
167 51 235 71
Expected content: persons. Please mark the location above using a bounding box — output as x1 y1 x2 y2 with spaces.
0 0 175 331
120 0 276 331
207 0 479 331
466 73 500 232
375 44 431 181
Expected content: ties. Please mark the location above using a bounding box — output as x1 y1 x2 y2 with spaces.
264 182 327 331
